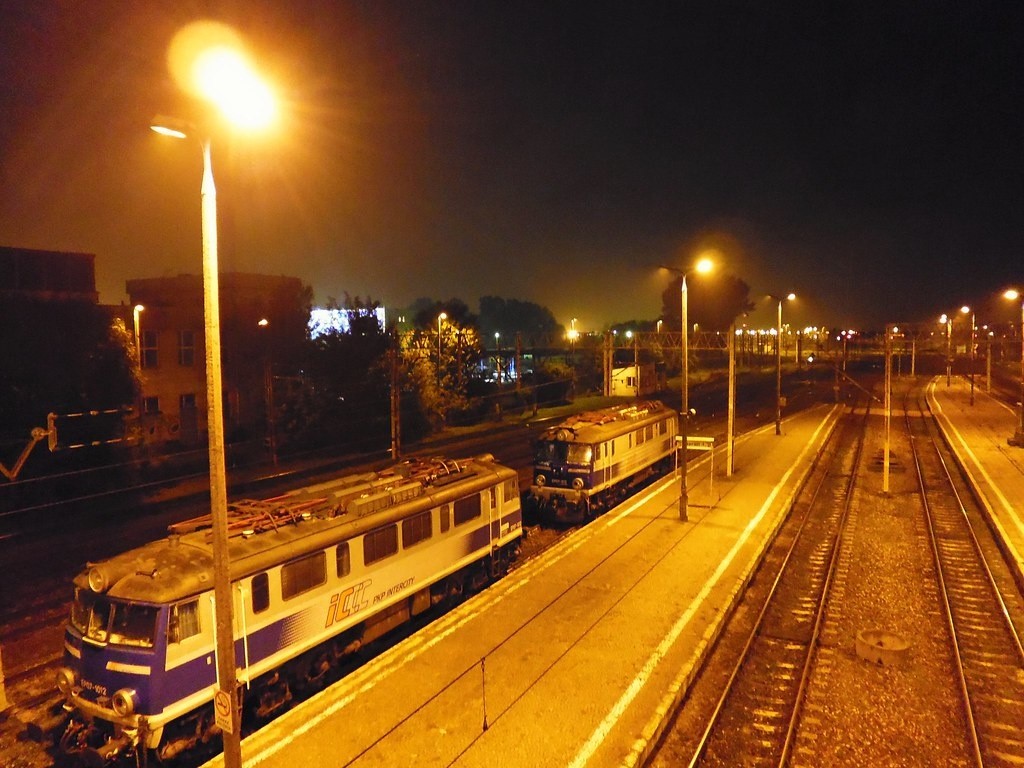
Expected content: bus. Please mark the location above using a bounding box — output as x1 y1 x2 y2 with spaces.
55 453 523 764
529 400 677 519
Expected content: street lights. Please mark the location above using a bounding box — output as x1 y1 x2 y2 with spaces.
658 258 715 520
496 332 500 354
570 316 578 369
766 293 795 435
940 315 952 384
962 307 975 405
1006 291 1024 446
437 313 446 384
149 121 314 767
